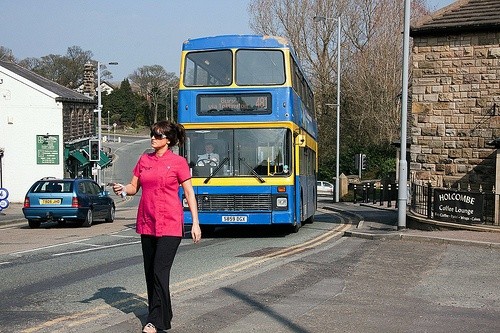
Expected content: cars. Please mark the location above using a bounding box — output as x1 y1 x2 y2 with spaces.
316 180 334 196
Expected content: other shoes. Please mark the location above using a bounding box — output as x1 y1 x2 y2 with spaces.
142 321 157 333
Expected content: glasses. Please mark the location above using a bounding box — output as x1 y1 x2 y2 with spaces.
150 132 167 140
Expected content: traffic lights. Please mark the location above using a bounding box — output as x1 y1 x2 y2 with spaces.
89 140 101 161
106 147 116 168
361 154 369 169
353 154 360 171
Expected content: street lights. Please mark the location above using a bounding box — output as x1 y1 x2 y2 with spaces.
313 15 341 203
97 60 120 188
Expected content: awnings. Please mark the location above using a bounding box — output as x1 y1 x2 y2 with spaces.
70 146 111 170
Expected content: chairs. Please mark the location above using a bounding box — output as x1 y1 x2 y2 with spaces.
46 185 62 192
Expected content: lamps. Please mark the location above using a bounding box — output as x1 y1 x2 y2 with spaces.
44 133 49 138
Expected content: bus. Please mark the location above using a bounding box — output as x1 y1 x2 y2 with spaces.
169 34 318 233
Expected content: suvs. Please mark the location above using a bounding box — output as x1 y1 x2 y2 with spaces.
22 177 116 228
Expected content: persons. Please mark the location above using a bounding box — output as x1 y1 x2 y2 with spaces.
196 143 219 166
112 121 201 333
